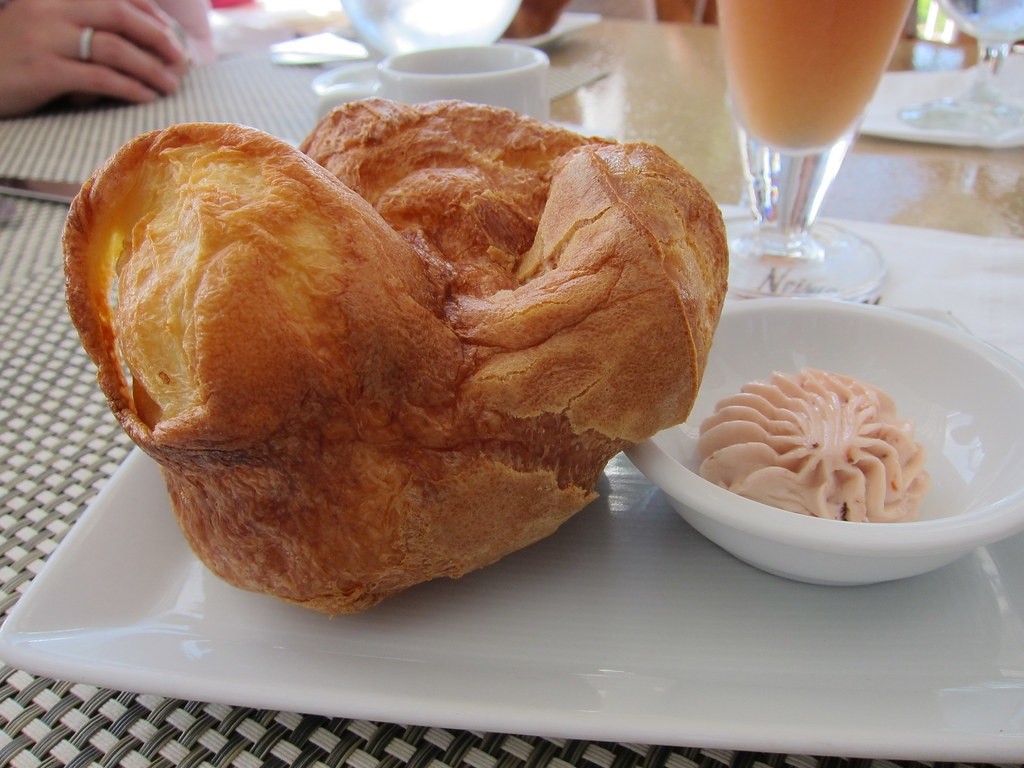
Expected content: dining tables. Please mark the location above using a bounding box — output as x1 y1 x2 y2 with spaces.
0 0 1024 768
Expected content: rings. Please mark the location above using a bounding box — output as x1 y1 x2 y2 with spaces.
79 27 96 61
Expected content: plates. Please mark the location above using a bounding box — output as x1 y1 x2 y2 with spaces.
266 7 603 66
0 204 1023 763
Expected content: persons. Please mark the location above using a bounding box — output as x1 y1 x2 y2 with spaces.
0 0 215 126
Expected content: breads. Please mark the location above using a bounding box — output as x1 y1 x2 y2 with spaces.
56 78 733 620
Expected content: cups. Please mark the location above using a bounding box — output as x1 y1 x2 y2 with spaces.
335 1 519 55
378 44 551 124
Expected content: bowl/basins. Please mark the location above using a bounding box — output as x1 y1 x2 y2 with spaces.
619 303 1024 583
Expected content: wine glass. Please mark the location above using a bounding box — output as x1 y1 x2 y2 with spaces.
698 0 908 306
897 1 1023 137
